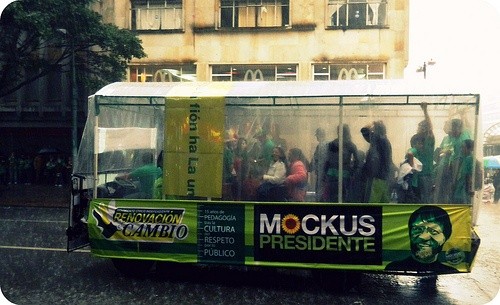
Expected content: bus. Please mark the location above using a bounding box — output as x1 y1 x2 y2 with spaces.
67 80 482 274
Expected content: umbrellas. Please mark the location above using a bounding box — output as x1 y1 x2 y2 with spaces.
483 155 500 177
38 148 57 153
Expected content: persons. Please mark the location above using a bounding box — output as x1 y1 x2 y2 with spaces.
492 169 500 202
116 153 162 200
157 103 475 203
0 153 69 188
